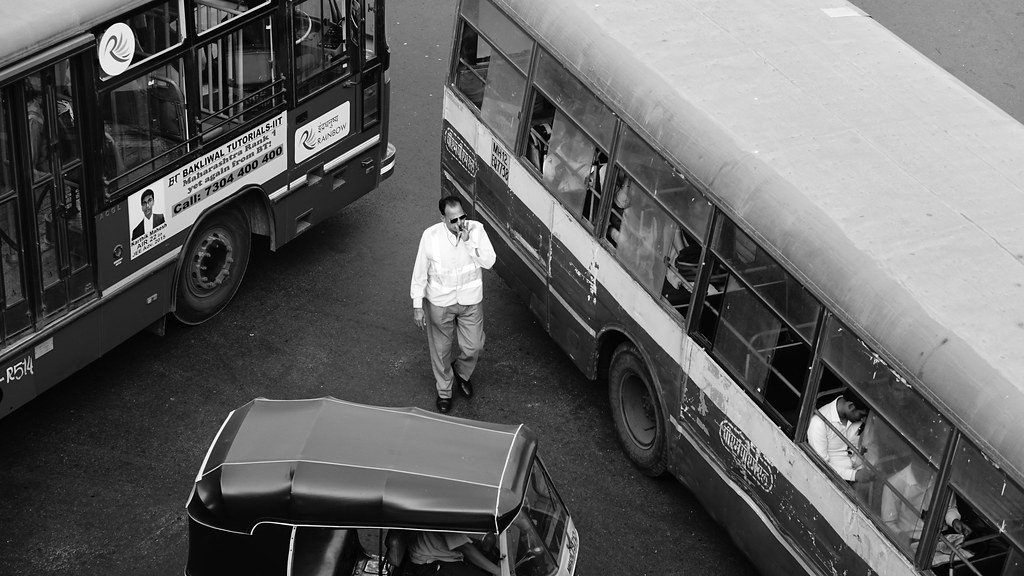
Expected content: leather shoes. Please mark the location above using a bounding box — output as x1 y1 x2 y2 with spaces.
437 395 451 413
452 361 473 397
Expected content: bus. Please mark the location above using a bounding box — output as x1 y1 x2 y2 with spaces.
0 1 396 419
435 2 1024 576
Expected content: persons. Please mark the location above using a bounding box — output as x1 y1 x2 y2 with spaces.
807 382 881 482
884 374 921 422
543 123 595 193
132 189 165 241
410 195 497 414
408 509 544 576
881 444 971 548
608 180 708 290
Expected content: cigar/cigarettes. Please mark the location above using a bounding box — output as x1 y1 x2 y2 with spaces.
459 225 463 231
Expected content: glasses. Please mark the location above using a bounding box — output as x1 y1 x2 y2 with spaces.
449 211 468 224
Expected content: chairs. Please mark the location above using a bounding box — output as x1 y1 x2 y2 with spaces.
45 76 223 195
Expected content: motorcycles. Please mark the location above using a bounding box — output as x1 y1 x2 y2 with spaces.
181 394 580 576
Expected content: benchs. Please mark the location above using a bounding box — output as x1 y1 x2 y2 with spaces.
455 68 994 576
378 528 410 576
292 526 368 576
